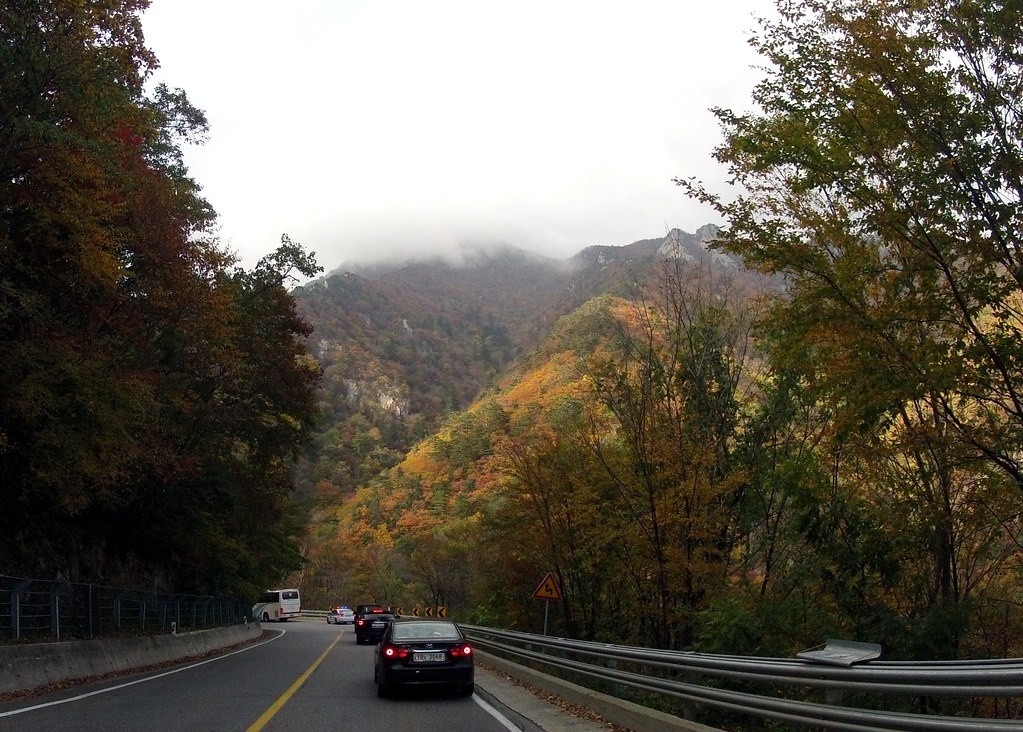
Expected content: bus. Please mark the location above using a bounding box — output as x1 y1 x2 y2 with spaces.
249 589 302 624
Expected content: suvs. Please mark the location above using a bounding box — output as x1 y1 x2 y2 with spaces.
352 604 382 625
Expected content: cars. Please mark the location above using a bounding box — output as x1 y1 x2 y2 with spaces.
373 617 474 699
325 606 356 625
355 611 399 644
